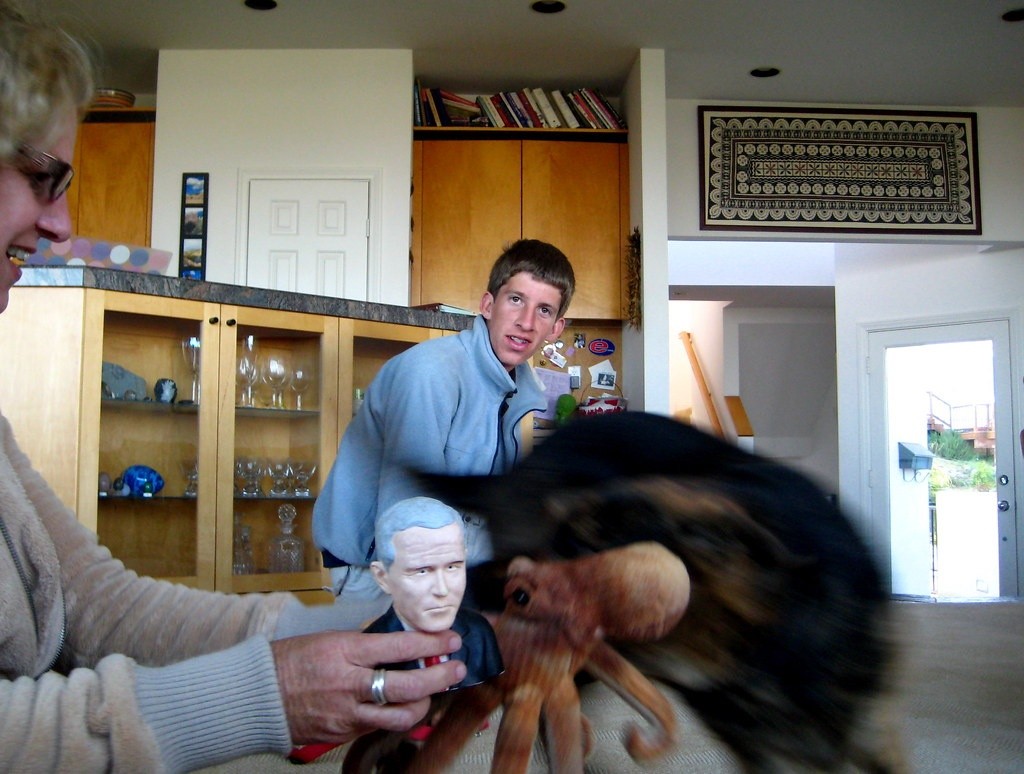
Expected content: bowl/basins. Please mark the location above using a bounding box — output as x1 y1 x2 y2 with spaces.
90 89 136 109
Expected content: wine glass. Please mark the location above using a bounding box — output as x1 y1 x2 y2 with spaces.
182 335 202 407
236 331 310 410
175 456 318 499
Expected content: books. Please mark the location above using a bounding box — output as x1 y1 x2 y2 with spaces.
413 75 629 130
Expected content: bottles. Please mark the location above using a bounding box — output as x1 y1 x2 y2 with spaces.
233 525 256 575
267 503 305 578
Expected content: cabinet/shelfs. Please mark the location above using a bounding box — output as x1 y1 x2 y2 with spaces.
65 109 157 248
413 128 630 320
0 264 469 602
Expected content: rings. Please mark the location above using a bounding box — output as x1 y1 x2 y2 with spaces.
370 670 389 705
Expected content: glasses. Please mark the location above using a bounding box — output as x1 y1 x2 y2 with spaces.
19 144 74 202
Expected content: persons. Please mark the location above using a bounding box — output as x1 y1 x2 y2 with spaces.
361 496 505 691
310 241 577 605
0 5 468 774
